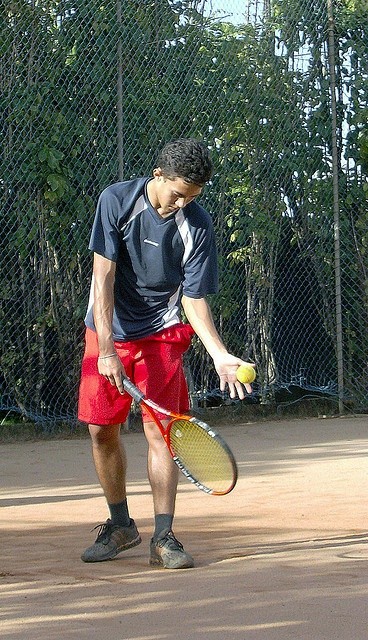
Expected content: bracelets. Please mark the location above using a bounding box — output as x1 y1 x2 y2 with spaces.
97 352 117 359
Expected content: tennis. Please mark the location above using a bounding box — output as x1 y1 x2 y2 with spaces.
237 366 256 384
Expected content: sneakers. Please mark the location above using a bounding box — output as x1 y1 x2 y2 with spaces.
150 528 195 569
81 517 141 562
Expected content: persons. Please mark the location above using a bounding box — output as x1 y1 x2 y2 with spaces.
76 137 257 570
